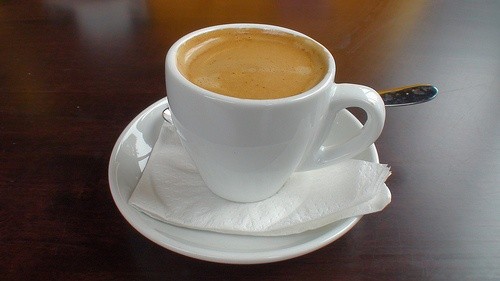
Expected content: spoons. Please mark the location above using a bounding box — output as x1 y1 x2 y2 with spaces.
161 83 438 126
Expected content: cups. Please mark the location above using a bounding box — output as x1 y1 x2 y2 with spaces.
165 22 387 203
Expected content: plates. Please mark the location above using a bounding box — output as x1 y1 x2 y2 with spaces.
108 98 379 265
